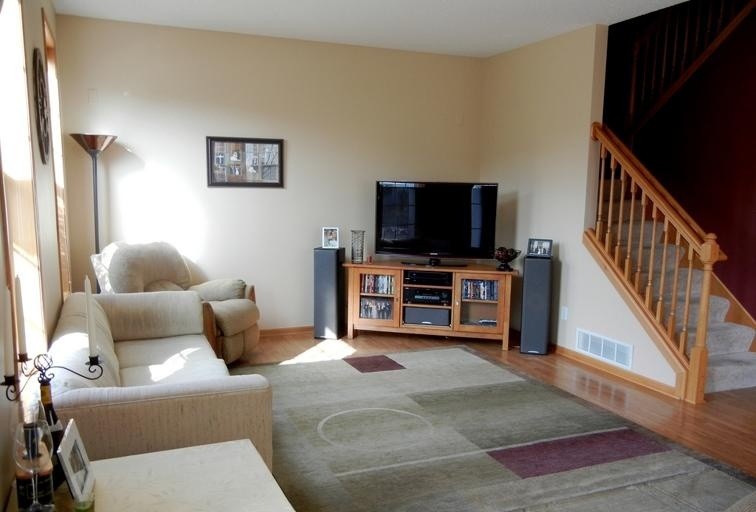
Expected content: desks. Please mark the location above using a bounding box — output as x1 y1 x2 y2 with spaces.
7 438 296 512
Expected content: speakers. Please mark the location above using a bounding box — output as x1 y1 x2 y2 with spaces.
520 256 553 356
313 246 347 340
404 307 450 326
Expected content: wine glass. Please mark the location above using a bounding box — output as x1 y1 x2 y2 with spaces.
13 422 56 512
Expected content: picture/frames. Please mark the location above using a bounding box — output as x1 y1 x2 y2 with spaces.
527 239 552 258
56 418 96 502
206 136 283 187
322 227 339 249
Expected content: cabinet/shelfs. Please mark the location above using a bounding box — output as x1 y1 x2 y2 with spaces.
342 261 519 351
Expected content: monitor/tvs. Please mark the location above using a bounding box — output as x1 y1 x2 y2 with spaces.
375 180 498 267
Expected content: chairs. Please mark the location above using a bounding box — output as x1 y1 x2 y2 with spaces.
90 241 260 365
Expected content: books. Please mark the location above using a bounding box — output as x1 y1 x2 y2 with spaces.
360 273 395 322
463 278 498 326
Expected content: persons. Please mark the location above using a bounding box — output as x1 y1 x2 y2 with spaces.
535 241 546 254
327 232 336 247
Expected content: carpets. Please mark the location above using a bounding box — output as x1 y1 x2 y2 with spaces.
228 344 756 512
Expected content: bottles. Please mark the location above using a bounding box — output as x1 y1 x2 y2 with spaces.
37 382 64 442
16 424 54 509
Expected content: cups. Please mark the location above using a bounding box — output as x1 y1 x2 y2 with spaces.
10 399 46 436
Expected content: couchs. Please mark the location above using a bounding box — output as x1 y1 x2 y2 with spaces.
25 290 272 474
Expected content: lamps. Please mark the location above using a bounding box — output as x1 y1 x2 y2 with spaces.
69 134 118 293
